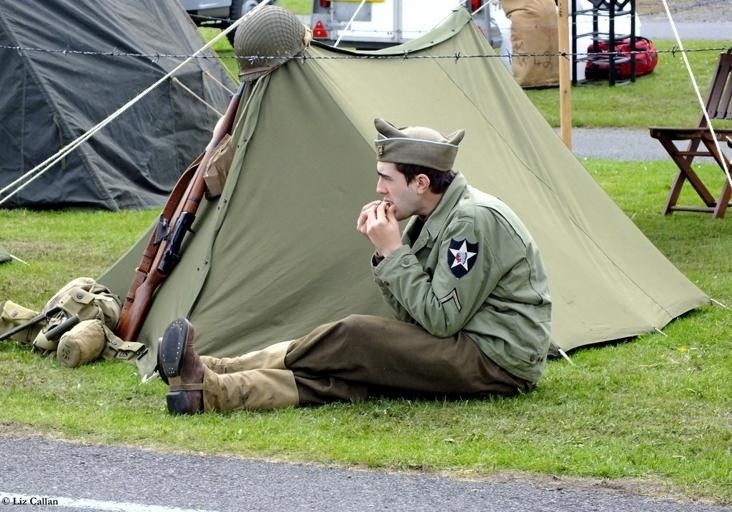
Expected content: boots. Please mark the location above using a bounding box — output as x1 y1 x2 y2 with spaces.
161 317 300 417
153 337 293 386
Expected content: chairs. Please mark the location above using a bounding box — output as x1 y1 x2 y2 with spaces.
650 49 731 219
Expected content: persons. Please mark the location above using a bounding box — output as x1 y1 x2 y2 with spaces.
157 118 552 416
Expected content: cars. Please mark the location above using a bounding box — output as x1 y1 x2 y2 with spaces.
176 0 276 48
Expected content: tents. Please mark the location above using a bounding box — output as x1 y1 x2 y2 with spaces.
94 4 730 365
0 0 242 212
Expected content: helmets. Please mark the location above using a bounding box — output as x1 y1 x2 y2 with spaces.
234 4 314 82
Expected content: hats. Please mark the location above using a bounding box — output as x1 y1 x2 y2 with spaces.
373 118 466 172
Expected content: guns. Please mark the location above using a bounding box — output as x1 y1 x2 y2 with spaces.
114 82 244 342
